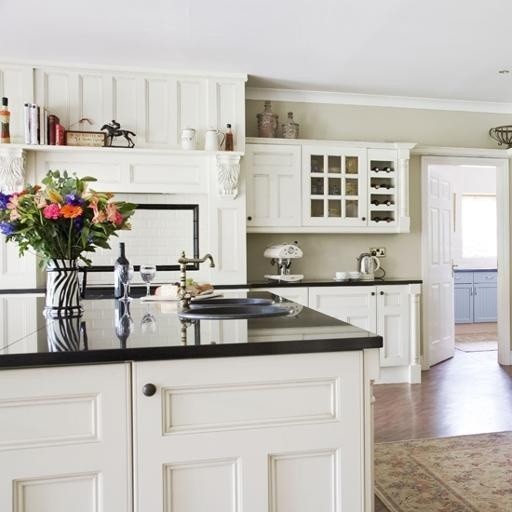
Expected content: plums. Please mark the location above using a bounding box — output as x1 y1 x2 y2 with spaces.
175 282 180 287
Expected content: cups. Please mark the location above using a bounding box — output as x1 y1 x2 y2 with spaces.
204 129 225 151
180 128 196 150
335 271 348 279
348 271 360 280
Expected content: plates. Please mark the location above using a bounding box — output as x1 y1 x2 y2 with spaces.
332 278 350 282
187 298 274 309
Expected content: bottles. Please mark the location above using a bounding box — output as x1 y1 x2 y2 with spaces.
224 123 234 151
255 99 300 139
113 242 131 299
0 97 11 143
114 303 131 350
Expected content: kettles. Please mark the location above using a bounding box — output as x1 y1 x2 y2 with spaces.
354 253 381 282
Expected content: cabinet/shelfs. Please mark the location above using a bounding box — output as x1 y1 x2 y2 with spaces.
301 141 368 234
309 283 421 384
131 348 366 512
248 285 309 308
368 143 416 234
1 361 132 510
242 139 302 233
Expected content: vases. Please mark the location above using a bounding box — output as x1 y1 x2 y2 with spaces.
256 100 279 138
41 258 85 317
44 314 80 351
281 112 300 138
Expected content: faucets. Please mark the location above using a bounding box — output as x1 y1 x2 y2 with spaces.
178 317 219 347
177 251 215 311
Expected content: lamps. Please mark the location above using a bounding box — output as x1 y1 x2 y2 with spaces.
487 68 512 150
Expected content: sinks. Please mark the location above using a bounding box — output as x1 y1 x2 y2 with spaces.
184 298 276 306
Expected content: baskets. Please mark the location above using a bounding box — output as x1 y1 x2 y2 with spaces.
489 126 512 148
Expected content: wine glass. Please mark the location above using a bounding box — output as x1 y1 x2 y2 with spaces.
116 264 135 301
141 301 159 337
115 301 136 337
139 264 158 300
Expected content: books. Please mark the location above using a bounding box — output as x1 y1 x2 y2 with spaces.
22 103 64 147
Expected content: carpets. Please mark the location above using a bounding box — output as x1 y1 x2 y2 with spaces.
373 429 512 511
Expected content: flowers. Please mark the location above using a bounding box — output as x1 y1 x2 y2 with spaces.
0 167 138 273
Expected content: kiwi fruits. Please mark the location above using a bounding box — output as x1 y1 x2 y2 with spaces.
195 284 212 292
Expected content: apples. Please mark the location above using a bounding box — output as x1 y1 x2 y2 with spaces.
186 278 193 285
